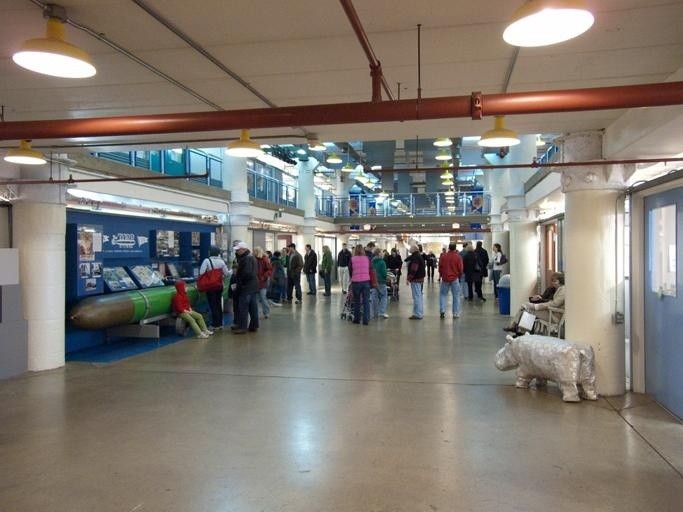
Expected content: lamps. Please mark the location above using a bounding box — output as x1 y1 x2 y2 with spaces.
502 0 595 48
477 112 521 148
438 160 454 169
435 150 453 160
444 188 456 212
11 3 97 80
442 178 454 186
308 140 414 218
226 128 260 158
3 137 49 166
432 137 453 148
440 170 454 179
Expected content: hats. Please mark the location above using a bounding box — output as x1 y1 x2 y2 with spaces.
233 241 248 250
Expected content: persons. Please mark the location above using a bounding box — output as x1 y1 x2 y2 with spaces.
228 263 238 329
266 240 489 324
200 246 232 330
254 246 273 318
503 273 556 336
489 244 503 301
512 271 565 338
231 242 259 334
172 281 213 339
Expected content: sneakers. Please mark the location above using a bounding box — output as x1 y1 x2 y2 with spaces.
407 314 461 320
230 325 257 334
208 324 224 331
204 330 214 335
378 312 390 318
196 333 208 339
502 324 524 339
294 299 302 304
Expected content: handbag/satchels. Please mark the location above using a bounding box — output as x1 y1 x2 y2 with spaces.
368 268 378 290
518 309 537 332
197 268 223 292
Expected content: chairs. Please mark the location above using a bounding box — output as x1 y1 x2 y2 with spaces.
534 303 565 338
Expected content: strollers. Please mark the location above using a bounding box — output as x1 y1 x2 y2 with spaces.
339 284 364 323
384 268 400 302
